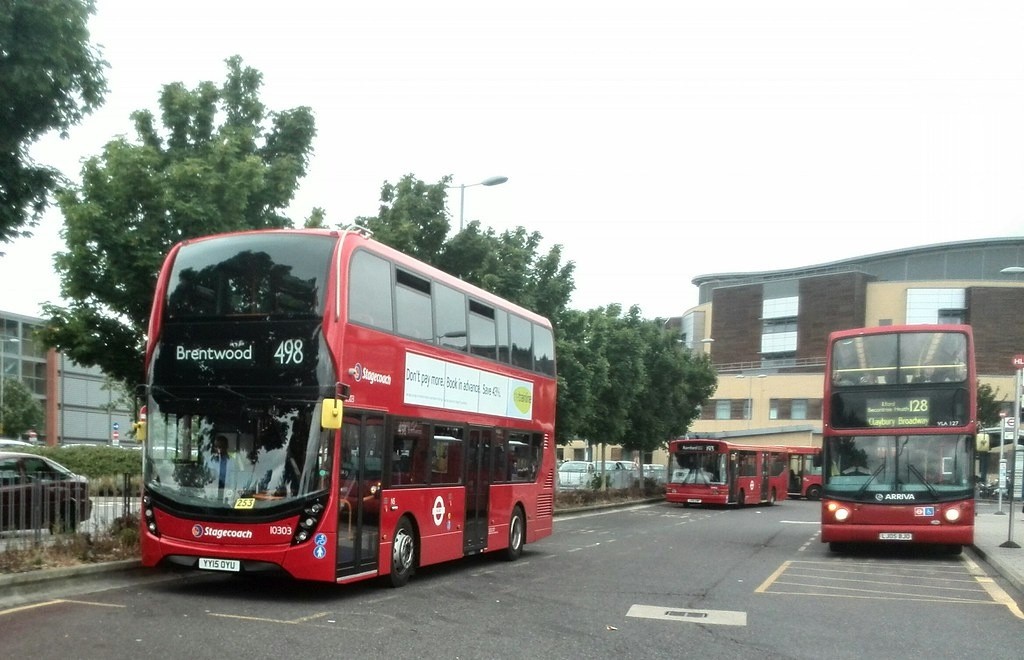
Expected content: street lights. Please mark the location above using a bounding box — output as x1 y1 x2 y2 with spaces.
456 175 508 278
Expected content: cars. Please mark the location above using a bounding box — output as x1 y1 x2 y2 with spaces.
0 450 93 536
132 445 182 454
59 443 96 450
555 460 666 501
0 438 38 449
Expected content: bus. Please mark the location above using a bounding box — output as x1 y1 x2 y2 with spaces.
812 320 981 554
785 443 825 501
136 225 559 590
662 438 788 507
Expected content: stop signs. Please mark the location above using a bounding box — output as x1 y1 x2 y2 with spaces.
29 432 37 439
139 405 149 422
113 430 120 439
